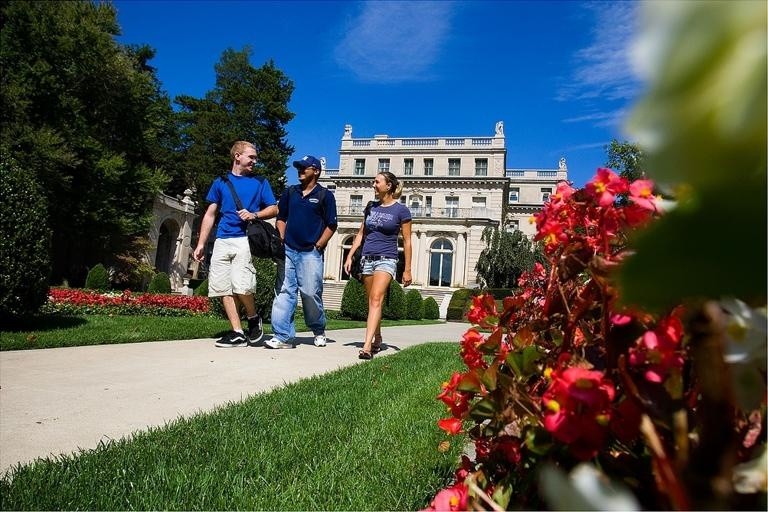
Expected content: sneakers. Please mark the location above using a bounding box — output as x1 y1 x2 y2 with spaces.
214 332 248 348
248 314 264 344
263 337 292 349
313 332 327 347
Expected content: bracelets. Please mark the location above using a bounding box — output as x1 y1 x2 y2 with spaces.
314 243 324 252
254 212 259 220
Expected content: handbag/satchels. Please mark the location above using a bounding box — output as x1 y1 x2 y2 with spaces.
348 200 374 284
245 219 281 258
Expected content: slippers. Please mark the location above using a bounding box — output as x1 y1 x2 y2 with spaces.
359 334 384 360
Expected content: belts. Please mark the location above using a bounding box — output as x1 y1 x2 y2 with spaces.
365 255 394 261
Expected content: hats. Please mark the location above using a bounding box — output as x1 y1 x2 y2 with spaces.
292 155 321 170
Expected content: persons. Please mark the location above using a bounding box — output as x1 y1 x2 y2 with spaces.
190 139 280 348
259 156 337 350
343 171 414 359
